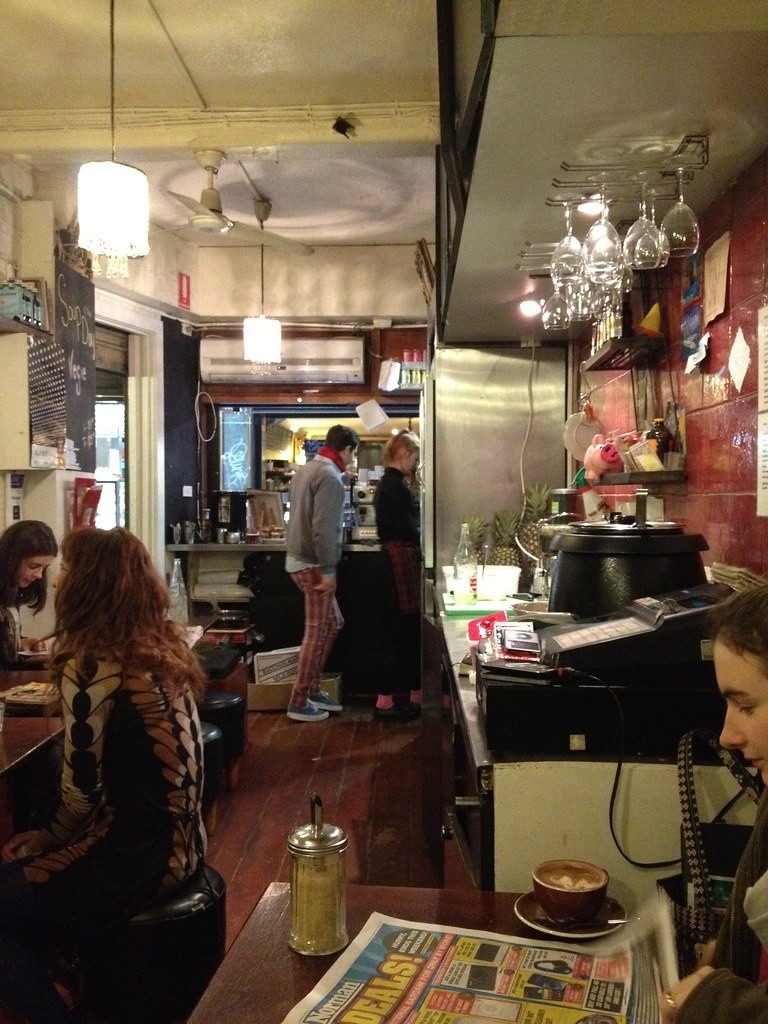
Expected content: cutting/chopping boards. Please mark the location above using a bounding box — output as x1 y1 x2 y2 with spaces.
442 593 540 617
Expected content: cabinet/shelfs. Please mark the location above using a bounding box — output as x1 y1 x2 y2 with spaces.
579 334 685 486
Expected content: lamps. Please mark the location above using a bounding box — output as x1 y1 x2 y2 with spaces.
78 0 151 278
244 201 281 375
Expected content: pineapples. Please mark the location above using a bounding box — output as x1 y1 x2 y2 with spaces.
461 481 553 575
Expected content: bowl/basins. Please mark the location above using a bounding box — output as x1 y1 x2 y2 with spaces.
442 565 522 601
358 465 384 485
214 609 250 628
532 859 610 925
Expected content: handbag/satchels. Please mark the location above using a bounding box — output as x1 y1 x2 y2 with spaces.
657 727 762 981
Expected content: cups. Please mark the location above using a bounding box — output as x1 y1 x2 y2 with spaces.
271 532 286 538
401 348 426 384
173 526 182 544
216 527 240 544
0 702 6 732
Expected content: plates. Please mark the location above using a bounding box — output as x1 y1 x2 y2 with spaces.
563 412 603 463
262 538 286 544
513 890 628 939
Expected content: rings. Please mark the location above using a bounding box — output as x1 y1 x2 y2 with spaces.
665 991 676 1008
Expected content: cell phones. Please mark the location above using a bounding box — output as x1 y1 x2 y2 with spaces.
18 650 50 655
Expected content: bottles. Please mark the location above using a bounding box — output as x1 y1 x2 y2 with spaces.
287 792 350 957
591 300 624 358
182 521 196 544
646 418 675 464
453 524 477 606
168 558 190 631
198 508 212 543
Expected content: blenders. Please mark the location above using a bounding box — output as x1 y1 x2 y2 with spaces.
529 488 578 594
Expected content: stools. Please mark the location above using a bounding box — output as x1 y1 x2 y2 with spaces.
195 645 248 714
69 865 228 1024
197 688 249 759
199 721 225 834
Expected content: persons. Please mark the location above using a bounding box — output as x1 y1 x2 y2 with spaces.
284 425 360 718
659 584 768 1024
370 429 422 720
0 520 58 666
0 526 207 1024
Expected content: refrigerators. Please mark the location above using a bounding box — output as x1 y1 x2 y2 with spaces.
418 344 568 861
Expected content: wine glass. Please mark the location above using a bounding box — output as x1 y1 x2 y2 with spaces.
541 154 700 329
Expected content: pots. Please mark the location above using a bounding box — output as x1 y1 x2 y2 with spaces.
547 487 711 620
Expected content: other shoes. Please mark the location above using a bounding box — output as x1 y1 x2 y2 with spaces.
307 691 343 711
408 700 420 712
373 701 420 721
286 698 329 722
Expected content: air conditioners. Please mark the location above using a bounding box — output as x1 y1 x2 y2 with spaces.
199 335 367 384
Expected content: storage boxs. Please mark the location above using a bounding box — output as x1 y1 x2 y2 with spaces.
248 645 343 710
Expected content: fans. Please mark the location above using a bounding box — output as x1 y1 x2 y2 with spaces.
151 149 315 256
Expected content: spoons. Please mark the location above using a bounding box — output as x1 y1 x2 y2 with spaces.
546 914 641 930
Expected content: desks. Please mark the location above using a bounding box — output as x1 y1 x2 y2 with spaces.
184 882 628 1024
0 616 220 846
